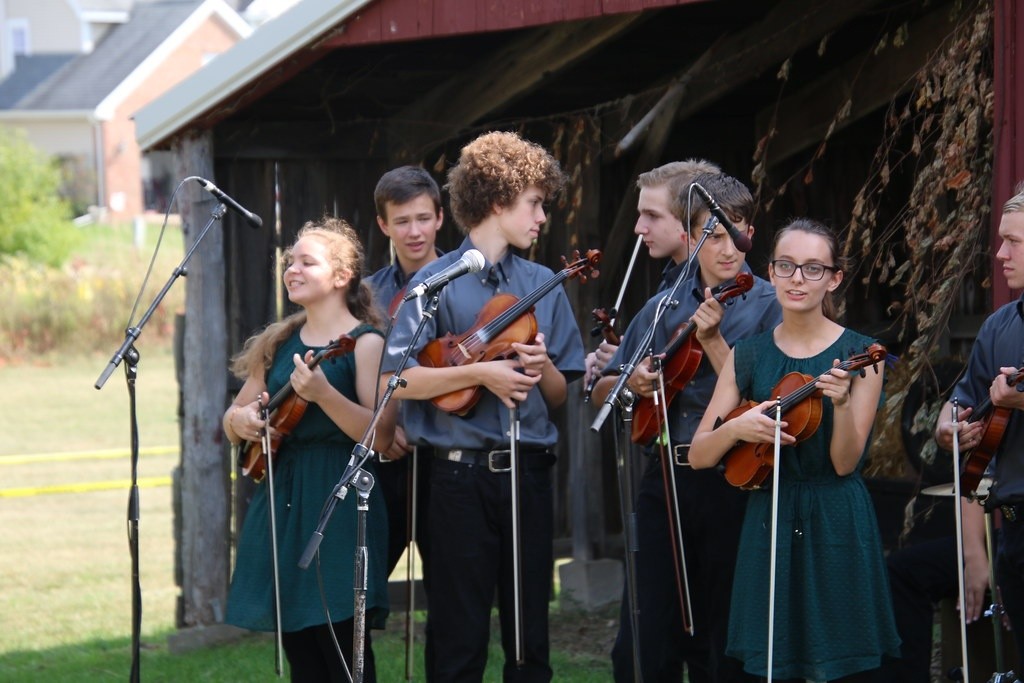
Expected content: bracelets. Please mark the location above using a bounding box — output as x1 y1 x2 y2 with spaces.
229 410 235 436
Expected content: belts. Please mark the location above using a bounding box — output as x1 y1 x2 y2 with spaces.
999 503 1024 522
433 447 558 473
651 443 691 466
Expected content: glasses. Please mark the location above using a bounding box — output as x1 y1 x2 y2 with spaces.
771 260 837 281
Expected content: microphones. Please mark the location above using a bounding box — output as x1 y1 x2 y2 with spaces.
196 177 263 229
403 249 486 303
694 181 752 252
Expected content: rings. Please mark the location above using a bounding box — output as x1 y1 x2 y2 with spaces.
256 431 258 437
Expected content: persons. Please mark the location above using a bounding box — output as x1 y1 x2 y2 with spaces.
935 191 1024 683
688 221 901 683
584 161 783 683
223 217 387 683
362 132 586 683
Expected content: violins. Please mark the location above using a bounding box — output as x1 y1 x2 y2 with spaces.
416 247 606 419
388 286 407 327
591 307 621 347
944 362 1024 501
712 342 889 495
236 333 358 484
630 271 757 448
